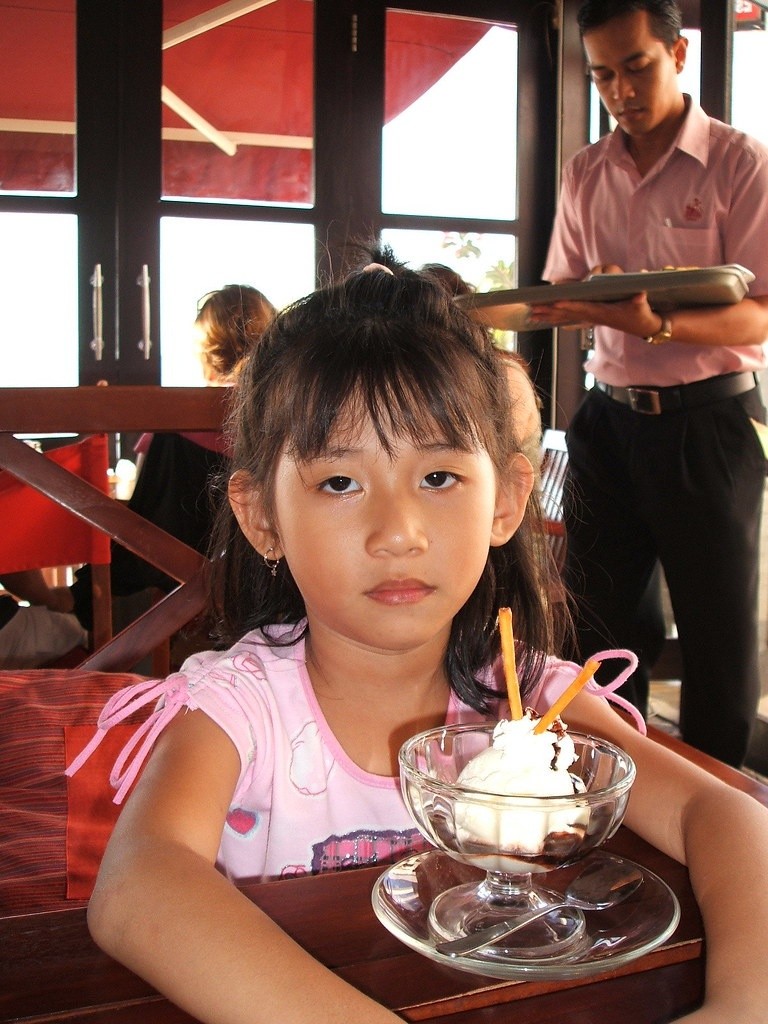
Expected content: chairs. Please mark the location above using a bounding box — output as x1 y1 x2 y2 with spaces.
535 427 571 585
0 431 111 670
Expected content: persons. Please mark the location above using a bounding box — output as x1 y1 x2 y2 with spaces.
523 1 767 771
1 247 766 1022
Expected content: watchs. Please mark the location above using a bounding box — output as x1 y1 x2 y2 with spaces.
643 308 672 345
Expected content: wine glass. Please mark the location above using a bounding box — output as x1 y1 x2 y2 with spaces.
396 721 639 961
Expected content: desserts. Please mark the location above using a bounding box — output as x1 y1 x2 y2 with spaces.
449 713 588 872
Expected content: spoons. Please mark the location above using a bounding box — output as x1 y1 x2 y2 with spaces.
434 855 644 958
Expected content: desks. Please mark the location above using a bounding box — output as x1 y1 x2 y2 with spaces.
0 813 768 1024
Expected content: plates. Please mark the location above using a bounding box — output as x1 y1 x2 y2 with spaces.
371 843 682 982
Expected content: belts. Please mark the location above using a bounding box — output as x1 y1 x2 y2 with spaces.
596 371 756 414
